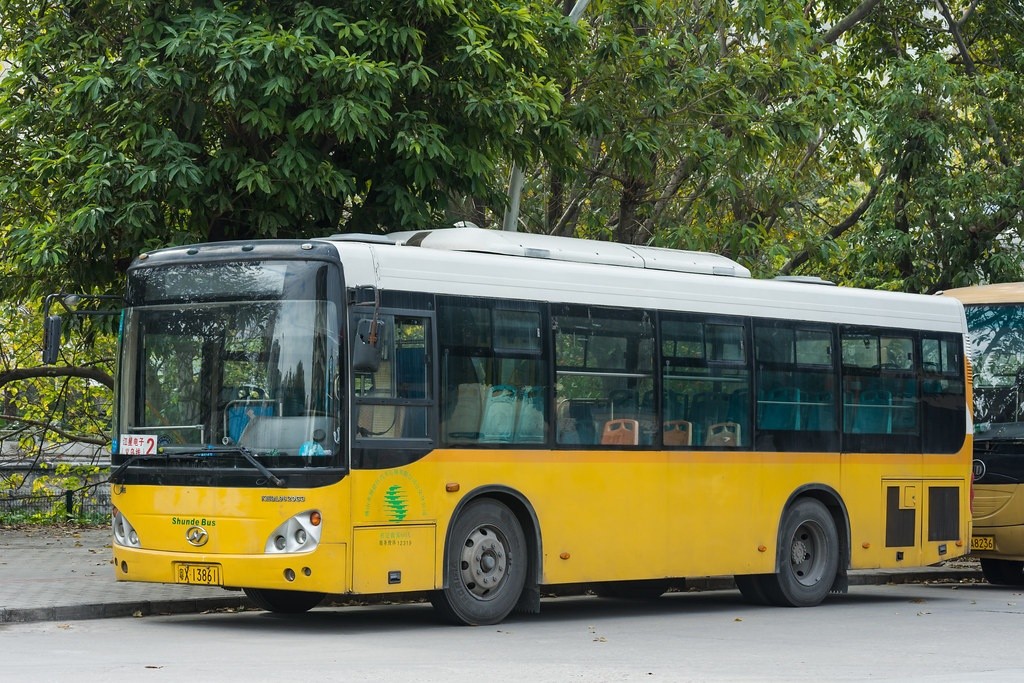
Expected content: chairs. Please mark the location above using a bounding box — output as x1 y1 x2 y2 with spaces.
359 363 943 445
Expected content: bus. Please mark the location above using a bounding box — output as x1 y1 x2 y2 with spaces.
39 223 983 629
927 281 1024 591
